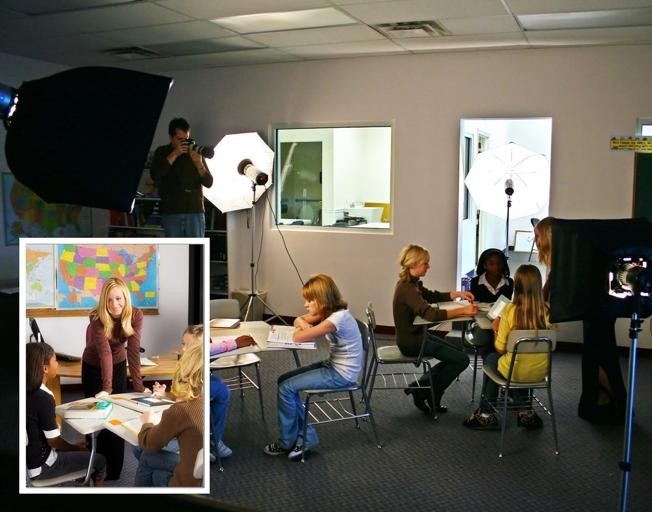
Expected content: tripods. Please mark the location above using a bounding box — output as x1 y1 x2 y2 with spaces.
237 186 291 327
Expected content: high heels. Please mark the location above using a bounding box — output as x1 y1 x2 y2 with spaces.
404 383 428 414
426 391 446 411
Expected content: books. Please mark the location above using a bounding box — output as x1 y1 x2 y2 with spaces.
111 204 144 226
438 299 470 310
477 302 497 311
487 295 514 320
65 401 113 421
267 325 318 349
210 317 242 328
131 395 174 407
124 413 162 436
213 334 255 349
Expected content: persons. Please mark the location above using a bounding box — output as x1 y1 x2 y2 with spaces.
149 117 213 238
395 245 471 416
138 325 204 488
22 342 106 486
533 218 586 302
263 274 363 459
210 336 256 464
85 278 151 482
465 249 515 356
464 264 546 431
153 326 203 399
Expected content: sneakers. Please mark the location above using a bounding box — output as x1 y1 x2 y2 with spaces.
288 442 310 459
518 411 542 427
211 440 232 458
264 442 291 455
210 453 216 463
464 409 497 431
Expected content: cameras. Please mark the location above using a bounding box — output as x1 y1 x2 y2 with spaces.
182 138 215 158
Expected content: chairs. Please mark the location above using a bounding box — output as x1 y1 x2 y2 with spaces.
482 330 560 458
210 299 265 421
300 318 382 463
359 302 440 423
456 321 483 403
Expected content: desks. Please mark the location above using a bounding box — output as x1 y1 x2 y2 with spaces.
209 321 317 368
413 301 489 325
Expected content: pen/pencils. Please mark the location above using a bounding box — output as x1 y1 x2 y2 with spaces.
471 299 475 304
